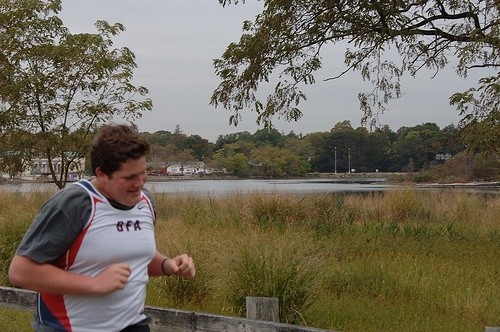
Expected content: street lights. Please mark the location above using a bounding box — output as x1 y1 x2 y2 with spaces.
347 148 351 173
334 147 338 173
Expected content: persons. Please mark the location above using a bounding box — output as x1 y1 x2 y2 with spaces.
7 123 196 331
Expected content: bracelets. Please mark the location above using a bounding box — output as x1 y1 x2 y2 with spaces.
160 258 172 276
